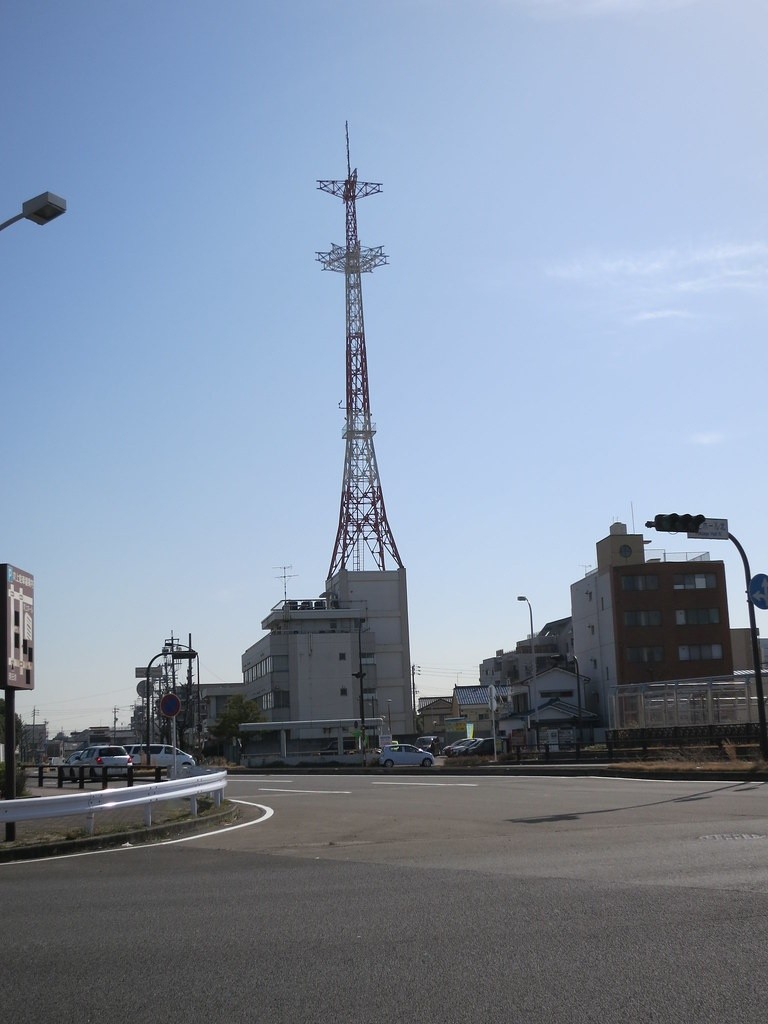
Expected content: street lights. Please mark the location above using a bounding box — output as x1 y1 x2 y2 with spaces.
146 650 196 766
359 618 367 765
387 697 392 733
166 645 201 753
551 653 582 756
517 596 540 758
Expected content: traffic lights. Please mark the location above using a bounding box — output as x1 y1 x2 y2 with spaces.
654 514 704 534
197 723 202 732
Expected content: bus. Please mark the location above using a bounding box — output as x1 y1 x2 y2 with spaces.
48 756 61 765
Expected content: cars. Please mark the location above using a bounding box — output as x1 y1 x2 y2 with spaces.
379 744 435 768
62 750 83 781
443 739 508 758
70 745 132 780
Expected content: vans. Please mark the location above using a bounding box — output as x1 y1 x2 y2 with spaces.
123 744 196 769
414 735 441 758
320 740 357 756
